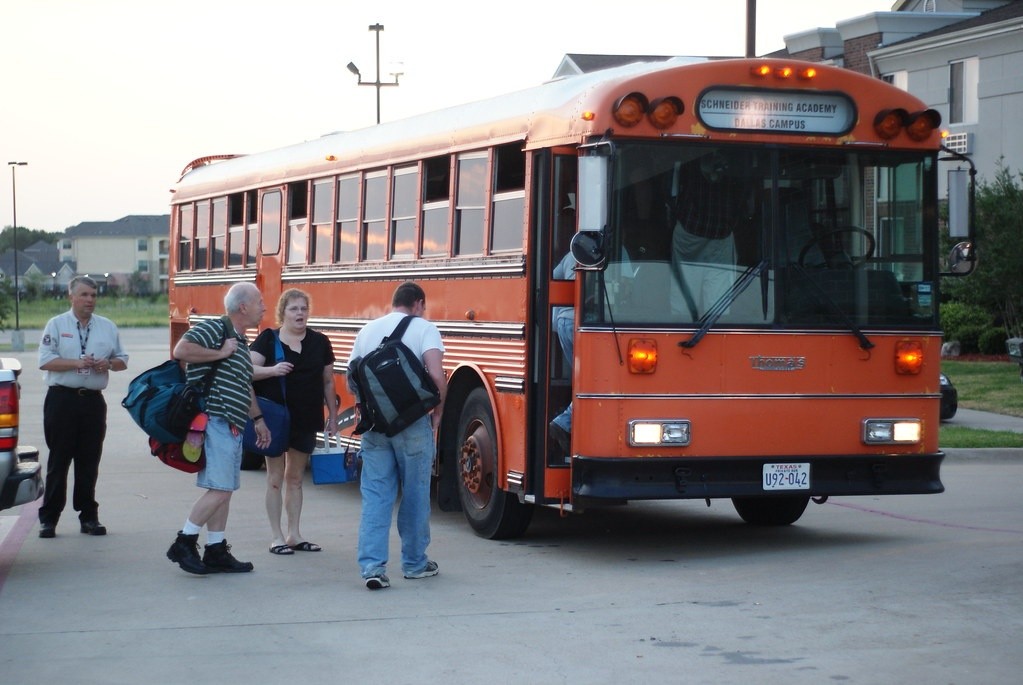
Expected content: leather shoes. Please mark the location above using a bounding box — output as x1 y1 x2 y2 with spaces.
39 521 56 537
80 520 106 534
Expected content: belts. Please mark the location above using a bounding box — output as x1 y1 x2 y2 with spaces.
49 387 101 396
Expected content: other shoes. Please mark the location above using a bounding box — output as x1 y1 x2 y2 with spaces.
550 421 571 454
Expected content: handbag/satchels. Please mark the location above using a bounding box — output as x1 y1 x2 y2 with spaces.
243 396 290 458
148 410 209 473
122 359 200 444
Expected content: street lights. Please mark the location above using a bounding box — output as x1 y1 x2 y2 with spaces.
9 161 29 328
347 22 405 125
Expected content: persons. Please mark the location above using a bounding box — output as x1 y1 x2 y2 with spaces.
550 156 824 455
163 282 271 575
248 288 339 555
38 276 129 538
346 282 449 590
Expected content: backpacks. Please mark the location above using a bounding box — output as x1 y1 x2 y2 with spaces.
348 315 442 438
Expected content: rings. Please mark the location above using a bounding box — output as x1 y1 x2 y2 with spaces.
100 367 102 369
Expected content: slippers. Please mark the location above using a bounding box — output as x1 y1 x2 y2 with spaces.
292 542 321 551
269 544 295 554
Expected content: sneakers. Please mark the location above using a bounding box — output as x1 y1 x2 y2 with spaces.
203 539 253 571
366 577 390 588
166 530 210 573
404 560 438 578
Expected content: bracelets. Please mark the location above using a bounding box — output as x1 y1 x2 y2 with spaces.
108 359 112 370
251 414 263 423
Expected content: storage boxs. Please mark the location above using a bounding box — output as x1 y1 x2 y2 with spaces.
311 431 360 484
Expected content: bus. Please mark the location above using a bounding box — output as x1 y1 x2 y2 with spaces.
169 55 981 542
